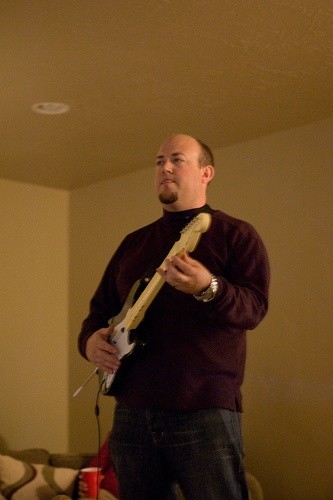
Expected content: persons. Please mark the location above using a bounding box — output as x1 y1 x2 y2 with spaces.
78 429 120 500
77 133 271 500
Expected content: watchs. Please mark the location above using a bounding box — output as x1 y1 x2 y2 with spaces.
192 274 219 302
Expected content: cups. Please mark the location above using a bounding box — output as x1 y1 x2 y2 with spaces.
81 468 101 499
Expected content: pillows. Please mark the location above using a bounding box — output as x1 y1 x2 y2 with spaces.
0 456 79 500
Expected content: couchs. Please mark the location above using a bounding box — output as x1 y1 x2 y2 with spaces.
0 446 263 500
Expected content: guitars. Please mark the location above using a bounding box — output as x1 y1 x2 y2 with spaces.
99 212 212 396
